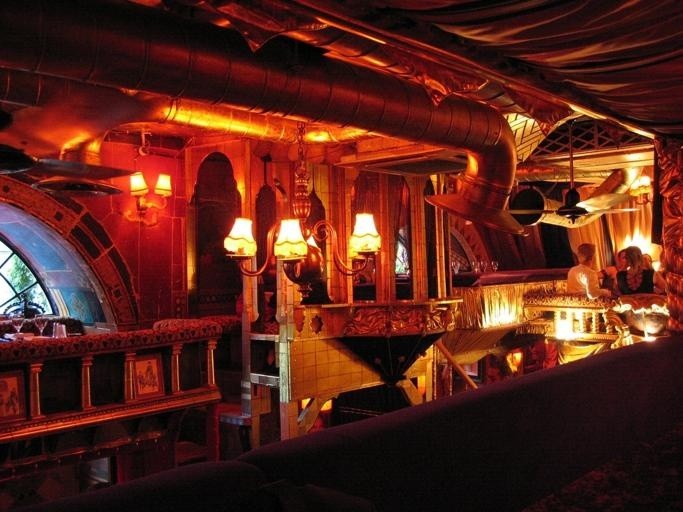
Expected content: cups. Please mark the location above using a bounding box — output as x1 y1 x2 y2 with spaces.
56 325 68 338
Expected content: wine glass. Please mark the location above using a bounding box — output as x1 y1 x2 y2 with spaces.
34 313 48 337
471 261 499 273
11 314 25 333
451 261 462 274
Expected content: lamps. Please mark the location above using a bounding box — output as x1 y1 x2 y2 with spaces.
129 169 174 222
220 120 382 298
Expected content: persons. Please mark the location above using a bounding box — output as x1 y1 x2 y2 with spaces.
567 243 611 300
597 250 626 293
262 289 277 371
614 246 666 294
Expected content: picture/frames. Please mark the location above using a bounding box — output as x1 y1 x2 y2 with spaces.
0 367 28 425
128 351 165 403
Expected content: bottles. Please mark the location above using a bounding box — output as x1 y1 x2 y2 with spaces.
52 322 59 339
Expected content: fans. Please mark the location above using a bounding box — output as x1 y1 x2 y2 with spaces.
507 125 641 226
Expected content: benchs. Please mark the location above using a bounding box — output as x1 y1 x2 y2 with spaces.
524 290 620 341
